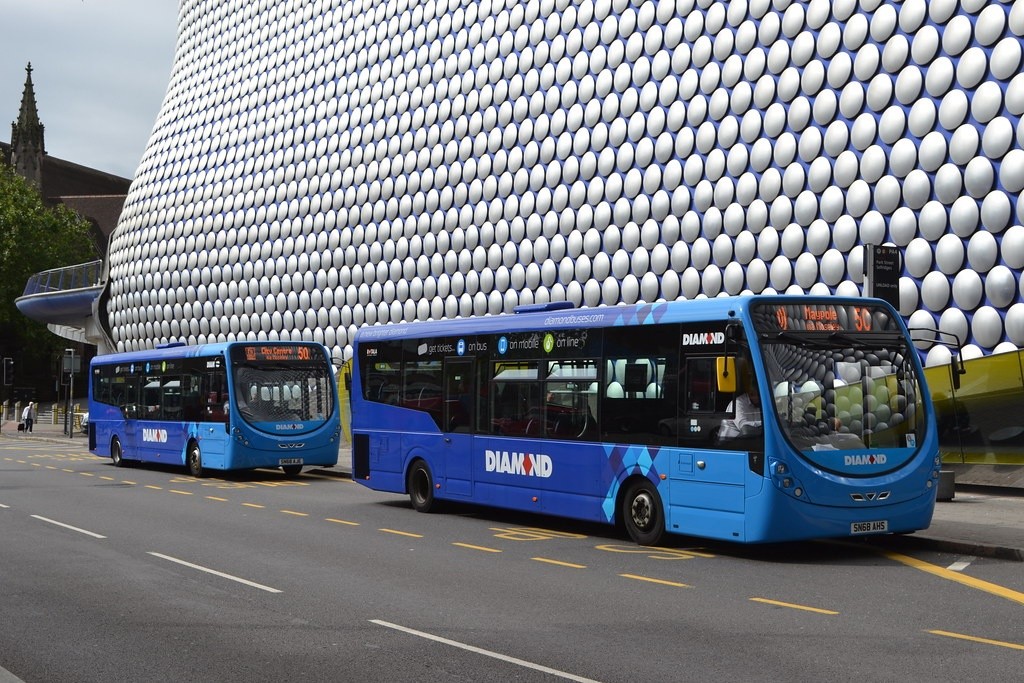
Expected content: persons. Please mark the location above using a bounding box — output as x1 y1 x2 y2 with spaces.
548 390 555 402
224 390 251 419
719 385 784 443
22 401 36 434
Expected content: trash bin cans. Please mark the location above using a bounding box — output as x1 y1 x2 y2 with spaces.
936 471 955 502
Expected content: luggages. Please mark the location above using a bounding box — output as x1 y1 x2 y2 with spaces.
17 424 25 433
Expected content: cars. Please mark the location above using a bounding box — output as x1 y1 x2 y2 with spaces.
79 380 199 439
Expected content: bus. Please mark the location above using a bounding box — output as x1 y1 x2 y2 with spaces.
88 343 352 478
349 293 967 548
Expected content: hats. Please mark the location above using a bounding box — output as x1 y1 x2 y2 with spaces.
29 401 33 405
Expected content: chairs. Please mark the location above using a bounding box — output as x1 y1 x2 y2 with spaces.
374 375 596 440
99 382 139 407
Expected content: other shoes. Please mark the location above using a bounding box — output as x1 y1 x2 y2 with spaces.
29 432 31 434
25 428 27 433
